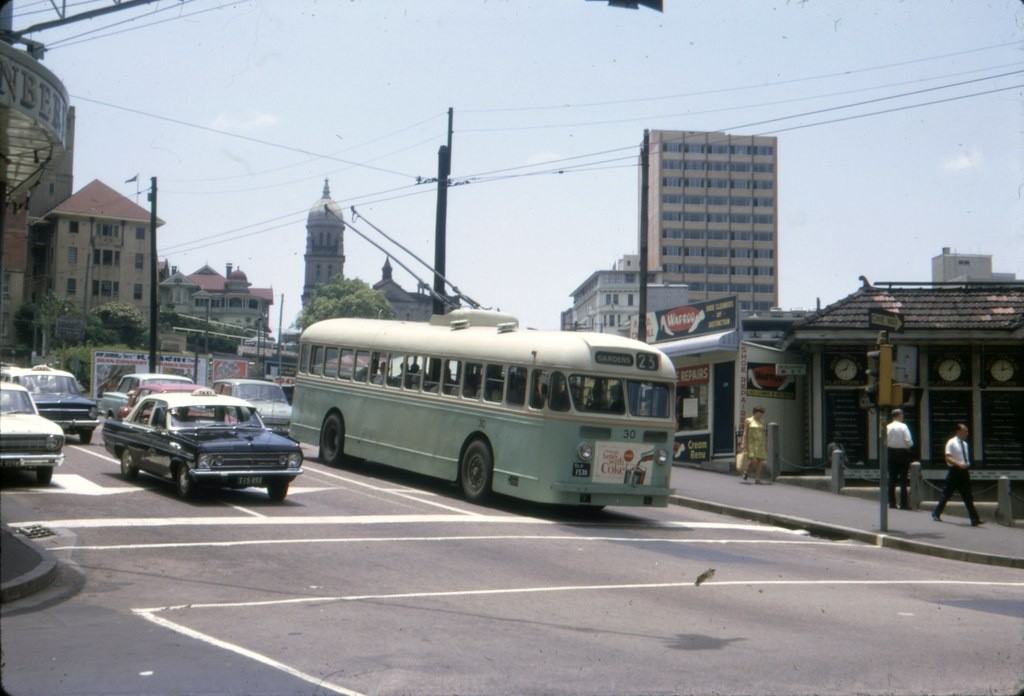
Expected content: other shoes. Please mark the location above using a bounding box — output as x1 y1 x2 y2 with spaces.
932 511 942 521
971 521 986 526
889 504 898 508
756 480 763 485
740 472 748 480
899 504 913 510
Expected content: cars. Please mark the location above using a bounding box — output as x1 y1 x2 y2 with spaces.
101 387 304 502
9 374 100 444
118 383 239 425
1 380 65 485
0 366 81 395
99 373 196 419
213 379 293 437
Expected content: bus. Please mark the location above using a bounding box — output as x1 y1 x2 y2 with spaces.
290 305 678 516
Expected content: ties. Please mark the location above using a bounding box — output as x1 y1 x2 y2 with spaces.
961 440 968 463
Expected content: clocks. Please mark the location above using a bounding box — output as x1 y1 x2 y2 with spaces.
834 358 858 381
939 359 962 382
990 359 1015 382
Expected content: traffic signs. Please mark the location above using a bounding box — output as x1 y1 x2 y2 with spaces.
868 308 908 331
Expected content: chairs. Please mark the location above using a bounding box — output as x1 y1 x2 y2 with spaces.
365 371 504 404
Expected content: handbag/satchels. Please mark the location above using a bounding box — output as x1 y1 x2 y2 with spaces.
736 448 748 471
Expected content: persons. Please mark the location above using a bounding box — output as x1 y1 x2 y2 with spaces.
932 424 987 526
588 382 626 414
887 409 914 511
176 407 191 421
742 406 767 484
184 369 193 378
356 358 580 412
213 408 225 421
